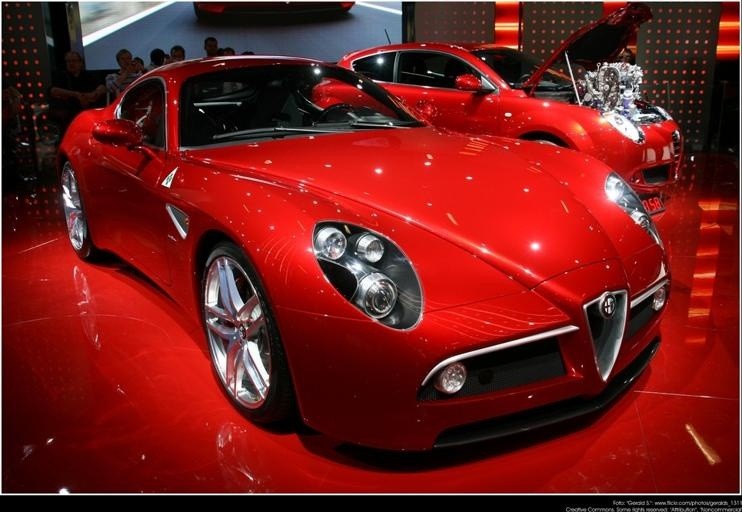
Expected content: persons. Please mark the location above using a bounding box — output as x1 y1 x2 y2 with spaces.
48 51 105 136
106 37 256 100
621 49 633 64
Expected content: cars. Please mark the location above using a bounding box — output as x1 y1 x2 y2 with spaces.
51 51 672 459
340 3 687 214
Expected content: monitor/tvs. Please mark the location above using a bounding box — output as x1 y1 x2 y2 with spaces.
66 2 414 92
